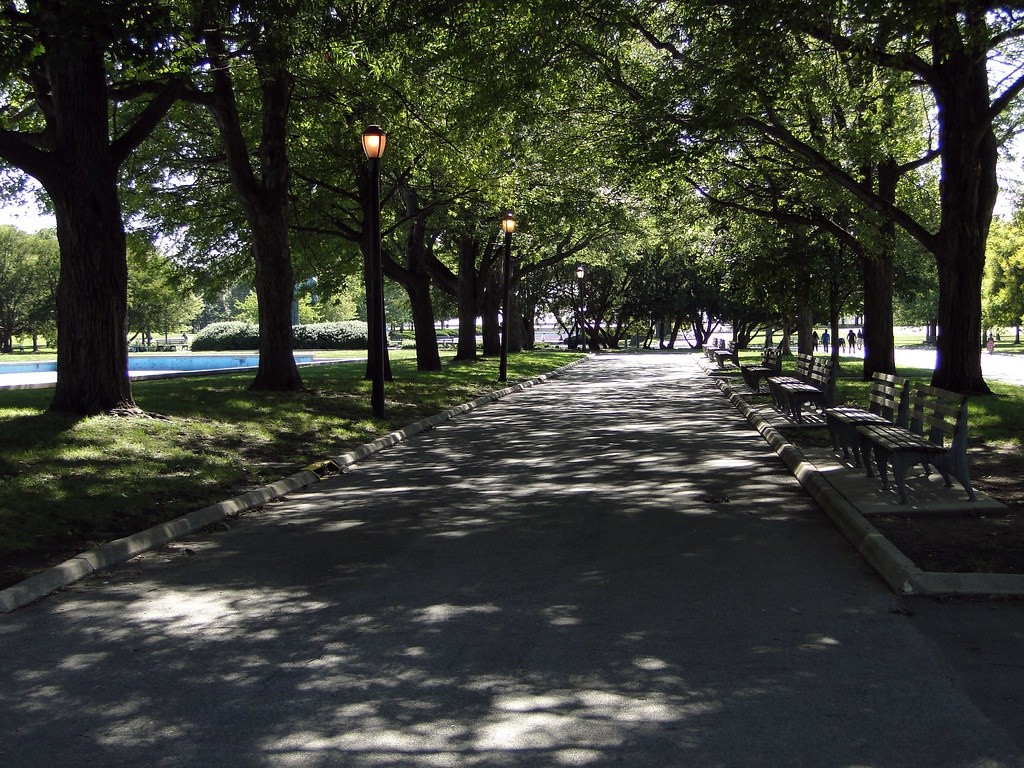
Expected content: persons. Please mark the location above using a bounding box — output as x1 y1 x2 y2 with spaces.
857 329 864 350
557 331 563 341
847 330 856 353
996 330 1000 341
987 334 994 354
821 329 830 353
812 331 819 351
839 338 845 353
181 332 188 351
541 332 546 342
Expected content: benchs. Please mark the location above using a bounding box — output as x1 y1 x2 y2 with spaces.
156 339 190 352
826 372 979 503
12 344 24 352
741 347 783 392
767 353 832 422
714 341 740 369
437 335 459 349
923 336 937 348
702 338 719 358
707 339 725 362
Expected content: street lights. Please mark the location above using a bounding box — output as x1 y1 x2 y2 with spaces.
358 124 387 421
576 266 587 353
498 211 516 380
